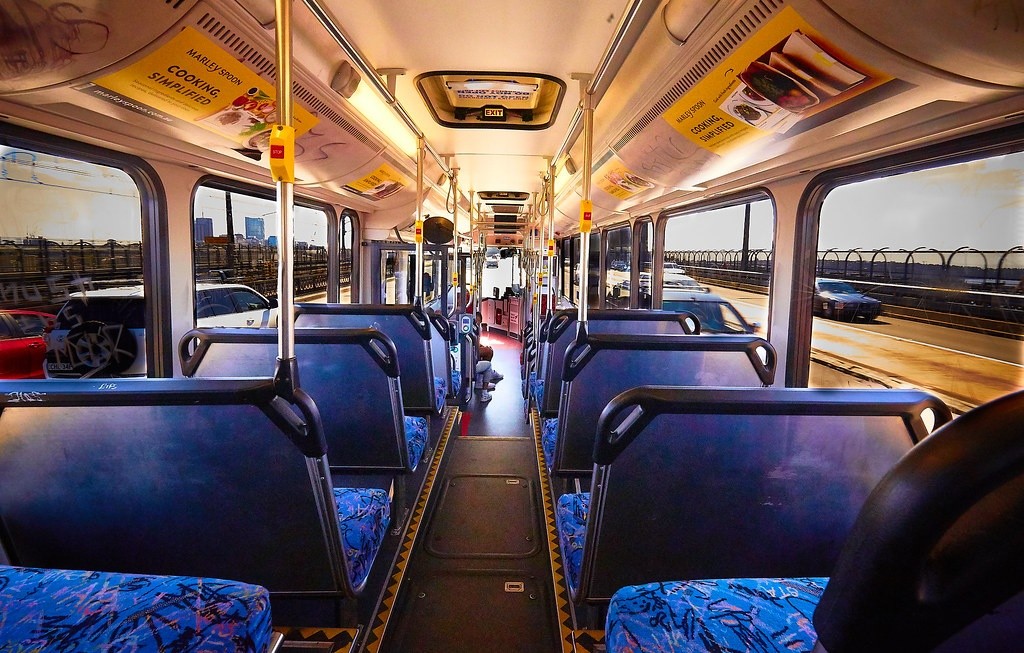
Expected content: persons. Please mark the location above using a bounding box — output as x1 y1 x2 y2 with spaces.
476 312 504 379
475 361 495 402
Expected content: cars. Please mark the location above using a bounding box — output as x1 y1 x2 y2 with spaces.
810 277 882 324
638 261 761 335
611 260 631 272
0 308 59 380
484 247 501 268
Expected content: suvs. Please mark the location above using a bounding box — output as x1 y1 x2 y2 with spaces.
42 283 278 380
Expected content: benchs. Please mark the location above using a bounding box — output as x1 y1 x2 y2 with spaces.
519 306 1024 653
0 301 480 653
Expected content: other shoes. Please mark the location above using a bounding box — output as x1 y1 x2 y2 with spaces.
480 394 492 402
476 381 496 389
490 371 503 380
